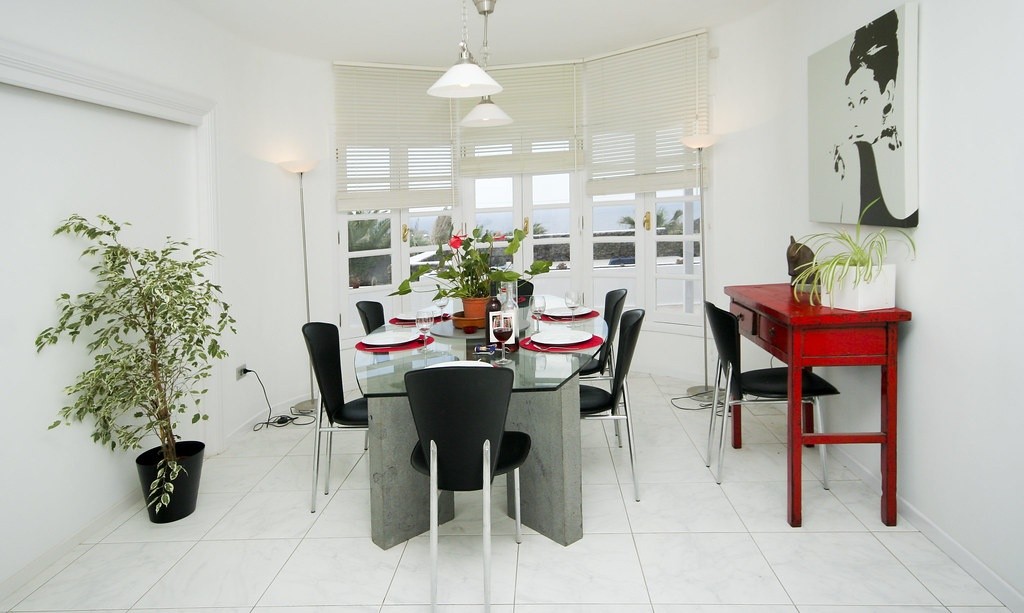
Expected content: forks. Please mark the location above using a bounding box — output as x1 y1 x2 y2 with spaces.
548 316 582 321
533 344 579 351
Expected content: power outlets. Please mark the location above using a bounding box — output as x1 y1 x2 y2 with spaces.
236 364 246 380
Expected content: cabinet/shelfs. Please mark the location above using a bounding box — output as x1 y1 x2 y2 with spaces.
723 284 913 528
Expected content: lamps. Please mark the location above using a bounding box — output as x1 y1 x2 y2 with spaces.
426 0 514 128
679 134 727 403
278 160 326 414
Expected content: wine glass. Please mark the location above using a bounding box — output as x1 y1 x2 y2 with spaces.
529 297 546 337
434 289 449 324
416 310 434 353
494 315 512 364
565 291 581 328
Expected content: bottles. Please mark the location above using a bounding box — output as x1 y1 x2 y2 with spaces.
496 283 520 351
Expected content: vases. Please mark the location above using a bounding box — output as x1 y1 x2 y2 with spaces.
462 297 491 318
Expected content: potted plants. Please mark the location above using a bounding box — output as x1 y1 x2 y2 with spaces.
34 213 238 524
789 198 918 313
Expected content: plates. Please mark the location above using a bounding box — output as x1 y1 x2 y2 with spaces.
531 330 593 344
361 332 419 345
542 307 591 316
423 360 493 368
396 313 441 320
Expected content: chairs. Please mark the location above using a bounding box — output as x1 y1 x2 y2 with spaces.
302 322 370 513
403 368 531 613
578 289 627 436
705 302 841 491
473 277 534 308
355 301 390 356
578 309 645 502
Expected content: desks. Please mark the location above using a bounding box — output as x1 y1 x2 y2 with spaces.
354 294 608 551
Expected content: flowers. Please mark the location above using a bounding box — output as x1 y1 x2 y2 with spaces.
387 225 554 303
